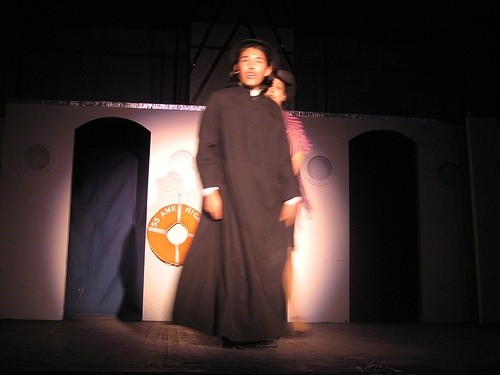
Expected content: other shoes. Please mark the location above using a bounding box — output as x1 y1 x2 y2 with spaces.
256 339 278 350
222 340 246 350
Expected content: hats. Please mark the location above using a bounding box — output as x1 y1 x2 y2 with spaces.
230 37 280 71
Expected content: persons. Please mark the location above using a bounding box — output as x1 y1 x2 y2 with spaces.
171 40 312 350
